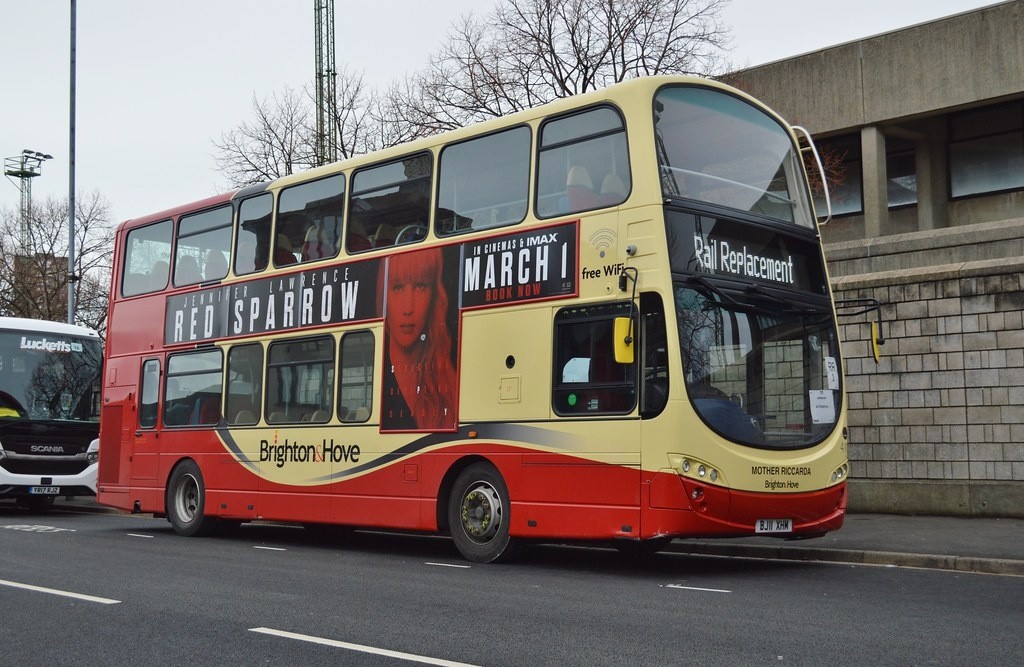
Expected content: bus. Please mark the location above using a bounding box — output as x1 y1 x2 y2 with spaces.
96 72 887 564
0 316 104 509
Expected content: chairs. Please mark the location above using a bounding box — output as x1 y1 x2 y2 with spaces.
700 165 751 212
599 174 628 208
127 219 419 301
567 166 602 213
660 164 692 198
189 397 370 424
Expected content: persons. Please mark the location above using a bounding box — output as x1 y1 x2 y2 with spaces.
376 245 459 431
